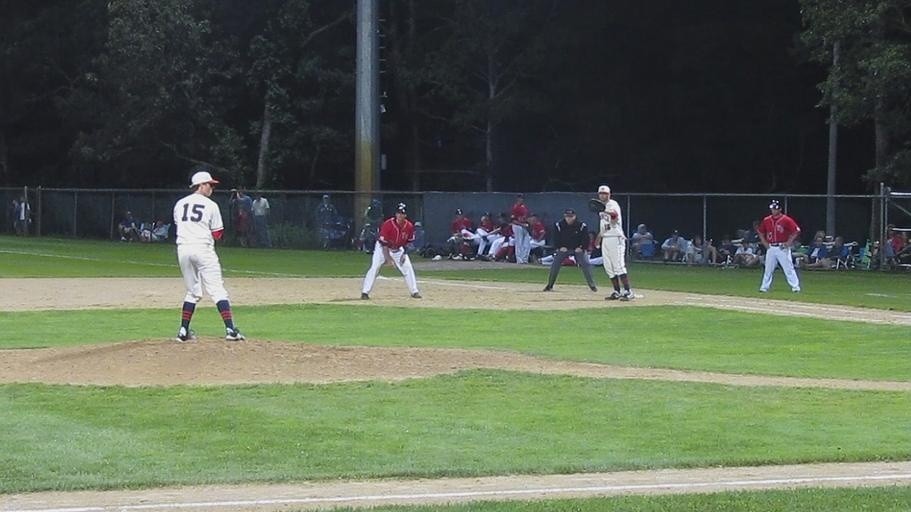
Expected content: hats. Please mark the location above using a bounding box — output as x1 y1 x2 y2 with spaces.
769 199 784 210
564 208 577 217
190 171 220 188
597 186 611 194
396 203 408 214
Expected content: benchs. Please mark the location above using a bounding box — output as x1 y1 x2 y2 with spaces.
795 241 858 270
882 264 911 272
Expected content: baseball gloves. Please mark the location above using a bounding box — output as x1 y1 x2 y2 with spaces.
589 197 606 212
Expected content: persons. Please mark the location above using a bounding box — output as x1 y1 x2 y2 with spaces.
359 199 423 300
589 185 635 300
757 200 801 293
431 195 602 292
315 195 339 249
119 211 171 243
8 196 32 236
632 224 911 272
228 185 253 248
251 192 272 248
172 171 245 342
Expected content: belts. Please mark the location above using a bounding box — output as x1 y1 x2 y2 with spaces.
770 243 779 246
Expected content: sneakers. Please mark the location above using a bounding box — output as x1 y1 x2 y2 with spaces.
760 287 768 292
225 326 245 341
792 287 800 292
411 292 422 298
361 293 369 300
590 286 598 292
175 326 199 345
543 285 553 291
605 289 636 301
